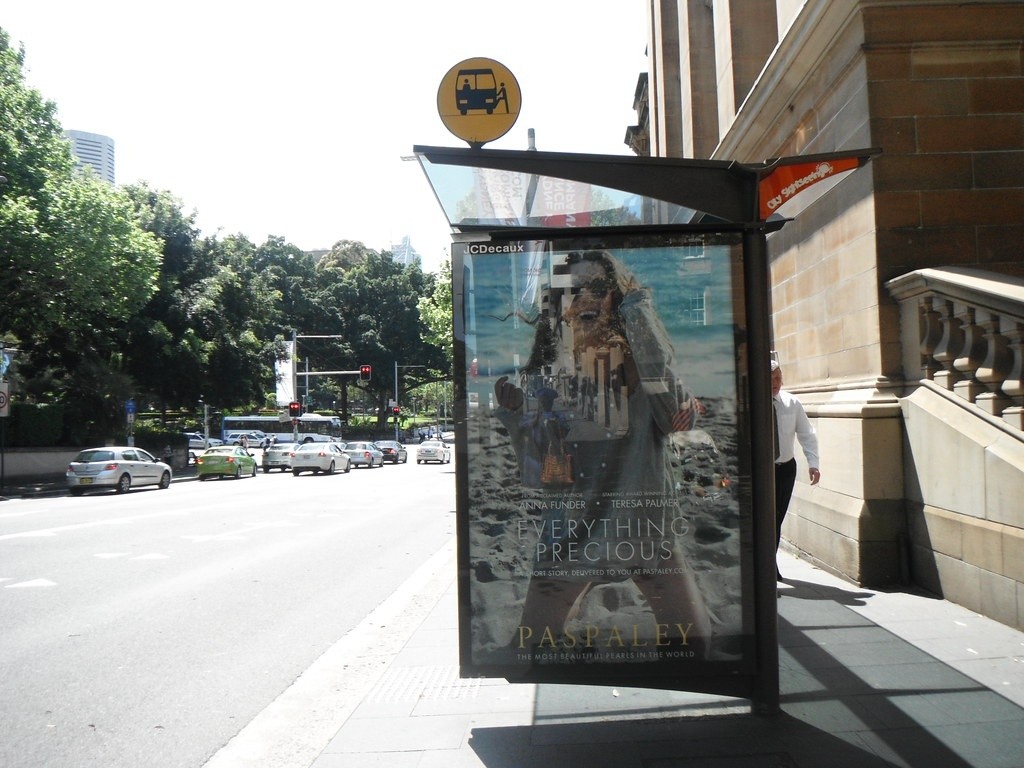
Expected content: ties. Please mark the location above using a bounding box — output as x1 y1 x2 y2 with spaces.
773 398 780 461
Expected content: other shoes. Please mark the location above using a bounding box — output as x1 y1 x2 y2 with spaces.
777 564 783 582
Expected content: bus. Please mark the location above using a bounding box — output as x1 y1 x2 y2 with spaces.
221 413 345 447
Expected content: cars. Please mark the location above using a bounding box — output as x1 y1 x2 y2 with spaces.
416 440 452 465
193 445 257 480
289 442 353 475
260 443 301 472
226 431 267 448
64 446 173 494
330 442 347 451
183 432 223 450
375 439 408 464
345 441 385 469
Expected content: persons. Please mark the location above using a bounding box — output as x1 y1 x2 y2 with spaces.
494 251 714 650
260 434 270 452
398 426 444 444
163 442 174 466
270 434 279 447
239 435 248 451
770 361 820 598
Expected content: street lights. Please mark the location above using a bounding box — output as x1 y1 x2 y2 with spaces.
391 362 426 441
292 329 342 441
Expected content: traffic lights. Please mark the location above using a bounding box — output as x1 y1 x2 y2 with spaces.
360 364 371 381
289 402 301 419
393 406 399 417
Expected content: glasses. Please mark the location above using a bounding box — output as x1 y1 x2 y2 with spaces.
565 278 613 302
